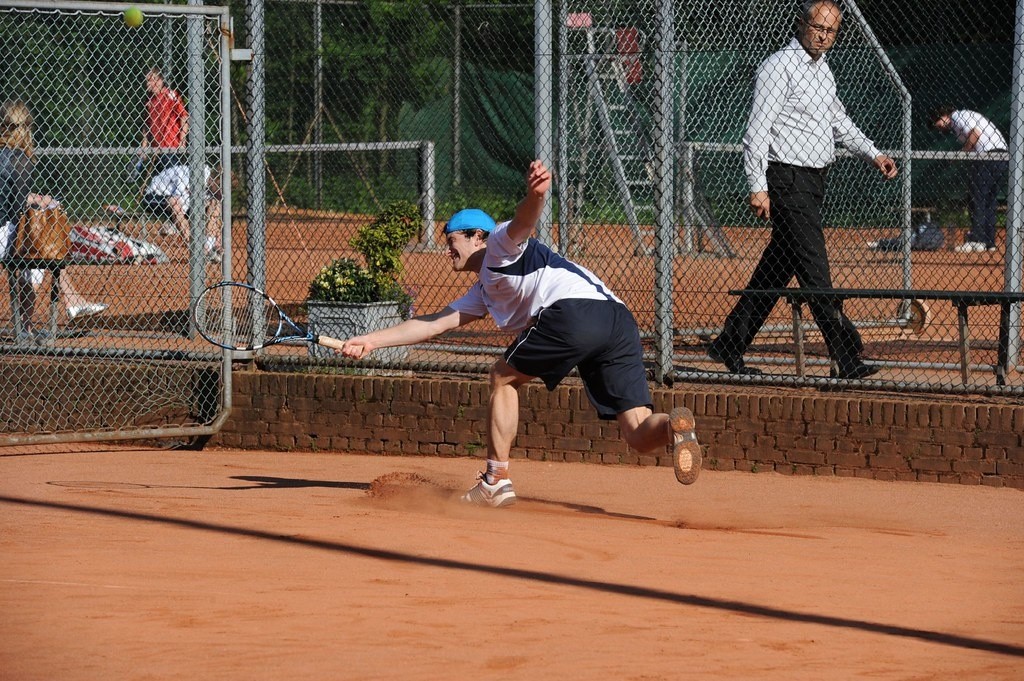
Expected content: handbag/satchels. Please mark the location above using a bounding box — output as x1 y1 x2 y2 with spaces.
14 203 70 262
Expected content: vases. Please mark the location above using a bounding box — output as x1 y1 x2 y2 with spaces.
306 300 412 364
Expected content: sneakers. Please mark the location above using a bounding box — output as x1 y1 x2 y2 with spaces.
461 470 517 508
670 406 703 484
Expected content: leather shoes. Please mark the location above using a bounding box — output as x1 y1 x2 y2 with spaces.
709 342 765 375
829 354 882 379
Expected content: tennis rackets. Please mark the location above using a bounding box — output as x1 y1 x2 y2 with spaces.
193 282 365 355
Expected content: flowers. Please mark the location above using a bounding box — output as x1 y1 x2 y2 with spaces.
307 198 419 324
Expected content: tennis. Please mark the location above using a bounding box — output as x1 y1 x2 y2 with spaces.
121 8 145 27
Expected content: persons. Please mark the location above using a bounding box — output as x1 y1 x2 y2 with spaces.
132 69 189 179
932 108 1011 253
702 0 881 380
0 103 108 341
331 159 707 514
140 159 240 265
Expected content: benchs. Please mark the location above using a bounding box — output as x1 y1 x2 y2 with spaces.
0 257 114 348
884 205 1010 254
727 287 1023 386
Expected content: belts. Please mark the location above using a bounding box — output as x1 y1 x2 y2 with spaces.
770 160 832 176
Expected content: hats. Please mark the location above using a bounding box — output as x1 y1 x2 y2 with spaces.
445 210 496 232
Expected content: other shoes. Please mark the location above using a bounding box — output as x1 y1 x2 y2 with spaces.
66 301 110 320
